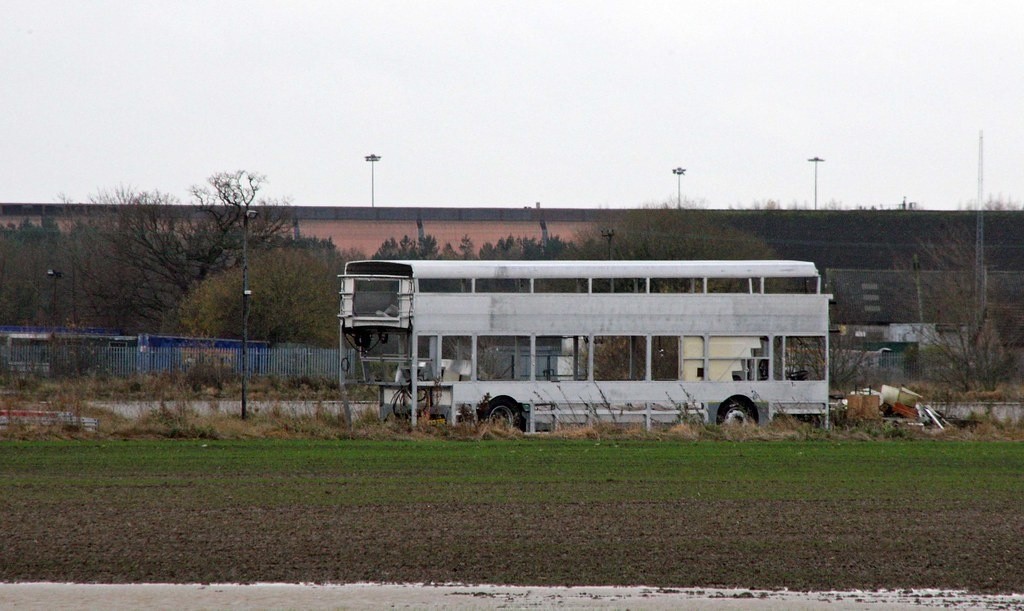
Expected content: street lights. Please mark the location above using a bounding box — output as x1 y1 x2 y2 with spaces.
671 166 688 208
808 155 826 210
46 267 60 414
364 152 382 261
238 208 260 422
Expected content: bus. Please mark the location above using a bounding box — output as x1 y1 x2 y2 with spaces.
335 255 830 434
